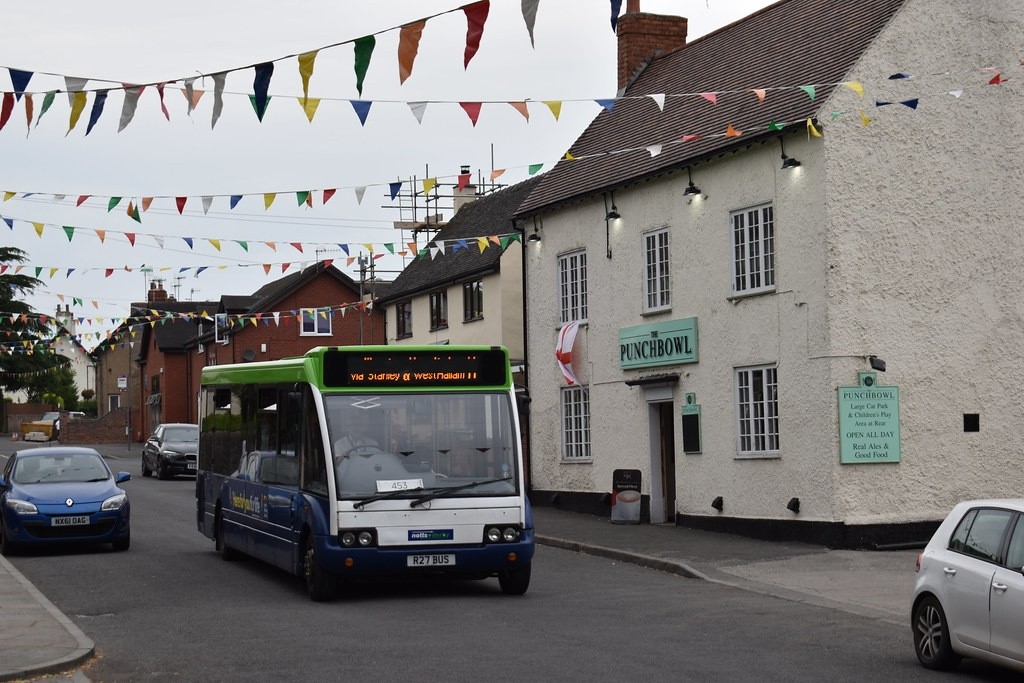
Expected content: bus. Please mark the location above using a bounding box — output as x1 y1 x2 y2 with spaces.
194 345 535 602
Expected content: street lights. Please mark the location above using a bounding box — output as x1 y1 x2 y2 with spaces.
86 363 95 414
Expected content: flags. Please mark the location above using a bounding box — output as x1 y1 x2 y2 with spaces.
555 322 580 385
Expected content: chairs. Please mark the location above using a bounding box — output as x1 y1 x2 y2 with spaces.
16 457 89 482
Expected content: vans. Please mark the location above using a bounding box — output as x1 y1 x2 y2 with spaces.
39 412 86 436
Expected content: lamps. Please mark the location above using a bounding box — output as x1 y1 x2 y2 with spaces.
683 181 700 196
604 206 621 221
782 156 800 169
864 354 886 371
527 226 541 242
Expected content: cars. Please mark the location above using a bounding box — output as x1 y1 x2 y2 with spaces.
247 450 299 492
0 446 131 557
141 423 199 481
909 498 1024 675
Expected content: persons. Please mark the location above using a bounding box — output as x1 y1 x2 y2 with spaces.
56 417 59 435
334 416 379 457
